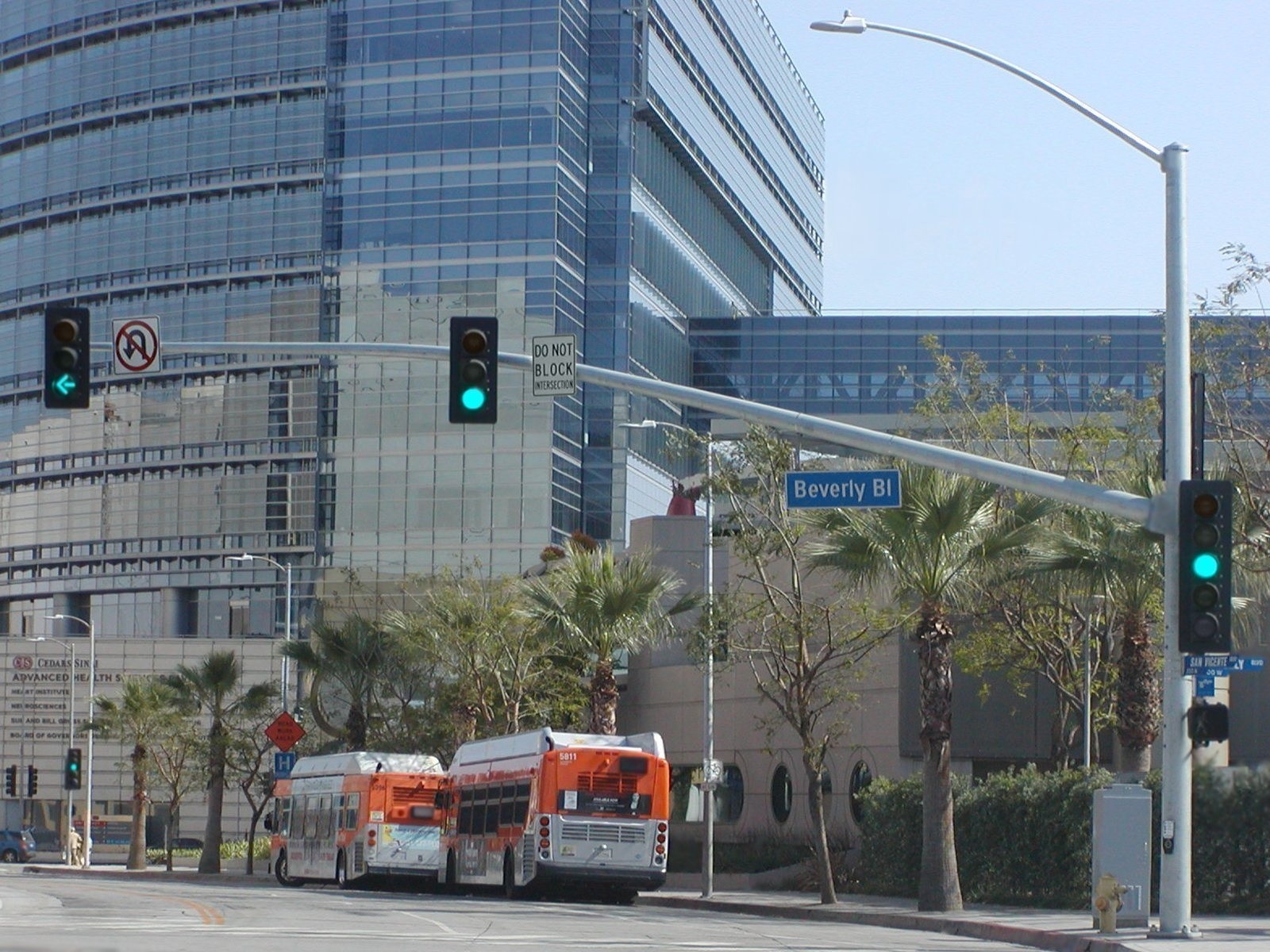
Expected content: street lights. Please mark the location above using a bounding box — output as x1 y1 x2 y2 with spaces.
617 419 716 899
42 614 96 869
805 14 1196 939
24 636 75 865
226 555 292 751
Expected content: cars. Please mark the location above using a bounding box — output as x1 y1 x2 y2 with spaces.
0 828 37 864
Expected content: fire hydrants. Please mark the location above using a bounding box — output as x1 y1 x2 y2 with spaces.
1094 874 1129 934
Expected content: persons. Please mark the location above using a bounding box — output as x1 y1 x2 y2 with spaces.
70 827 93 866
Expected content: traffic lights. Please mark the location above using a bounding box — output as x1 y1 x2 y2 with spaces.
28 765 38 797
1178 480 1232 654
5 764 16 797
449 315 500 424
1187 702 1229 742
43 304 90 409
66 748 81 790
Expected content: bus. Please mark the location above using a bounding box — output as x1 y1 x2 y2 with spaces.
434 725 670 906
263 750 445 891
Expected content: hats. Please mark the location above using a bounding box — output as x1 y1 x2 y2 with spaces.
70 827 75 831
